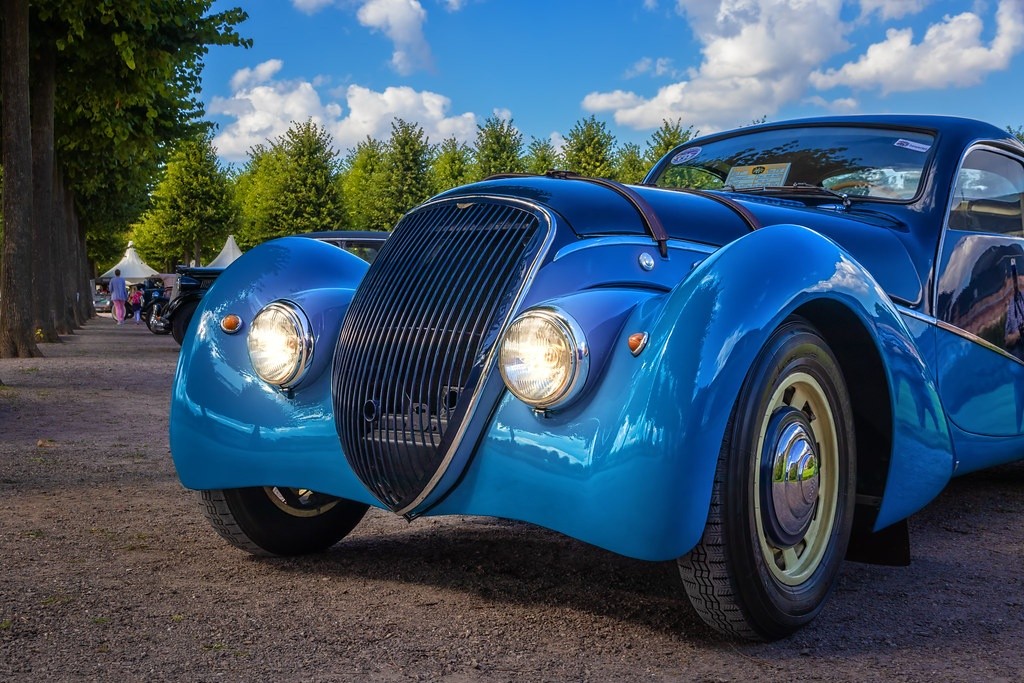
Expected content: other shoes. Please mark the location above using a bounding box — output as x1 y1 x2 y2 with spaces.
137 321 139 325
117 320 124 325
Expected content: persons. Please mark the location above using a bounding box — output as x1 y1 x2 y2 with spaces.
128 284 146 325
109 269 128 327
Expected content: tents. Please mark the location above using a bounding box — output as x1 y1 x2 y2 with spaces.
99 234 244 288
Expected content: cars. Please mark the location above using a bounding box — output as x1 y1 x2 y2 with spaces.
158 229 394 351
92 295 114 313
167 112 1024 645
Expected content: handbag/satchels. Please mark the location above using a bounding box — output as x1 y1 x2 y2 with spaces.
140 296 145 307
